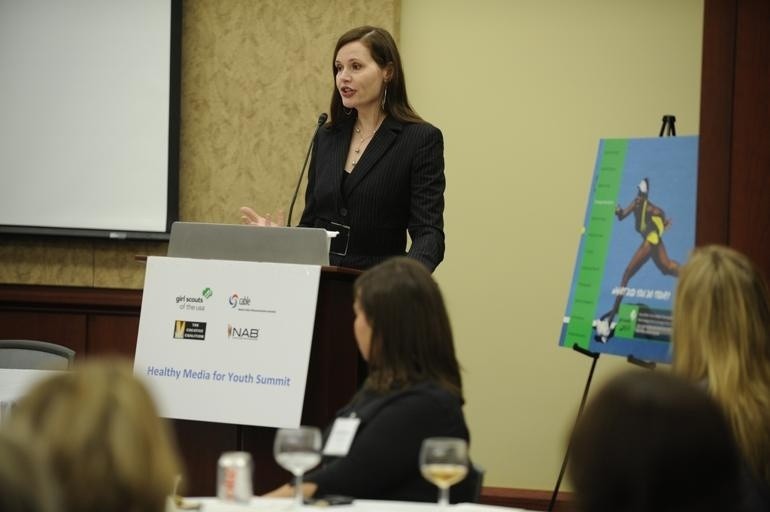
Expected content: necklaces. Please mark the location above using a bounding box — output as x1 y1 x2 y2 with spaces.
351 124 375 164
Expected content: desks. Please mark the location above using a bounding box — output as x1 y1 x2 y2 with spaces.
0 369 66 405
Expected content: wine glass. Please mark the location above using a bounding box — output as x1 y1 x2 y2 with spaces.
271 424 328 512
417 433 474 512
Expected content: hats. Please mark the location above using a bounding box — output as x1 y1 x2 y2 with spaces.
637 181 647 194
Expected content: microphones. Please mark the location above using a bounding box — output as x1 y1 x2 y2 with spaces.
283 109 331 226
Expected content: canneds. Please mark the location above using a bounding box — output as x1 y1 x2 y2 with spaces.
217 451 251 502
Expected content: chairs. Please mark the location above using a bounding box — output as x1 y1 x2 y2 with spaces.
0 340 77 370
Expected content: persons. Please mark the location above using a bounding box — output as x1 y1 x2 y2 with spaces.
560 367 769 510
261 257 481 505
0 355 181 511
241 26 446 274
667 243 770 461
590 177 684 335
